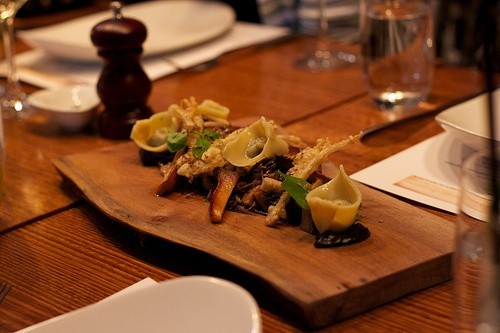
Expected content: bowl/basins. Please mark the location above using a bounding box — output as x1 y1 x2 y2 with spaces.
27 85 101 127
435 88 500 154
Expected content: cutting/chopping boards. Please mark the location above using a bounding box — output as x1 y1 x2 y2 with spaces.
50 140 454 329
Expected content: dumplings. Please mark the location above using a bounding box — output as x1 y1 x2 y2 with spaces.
130 111 179 153
305 163 363 235
221 116 290 167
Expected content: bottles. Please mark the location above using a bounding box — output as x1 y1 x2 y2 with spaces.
89 2 153 140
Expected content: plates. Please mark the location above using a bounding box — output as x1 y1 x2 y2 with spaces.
15 276 261 333
17 0 235 62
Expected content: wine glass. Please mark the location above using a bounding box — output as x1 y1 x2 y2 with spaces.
291 0 358 73
0 0 27 113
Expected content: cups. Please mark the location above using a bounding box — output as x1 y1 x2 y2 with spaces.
360 1 434 113
